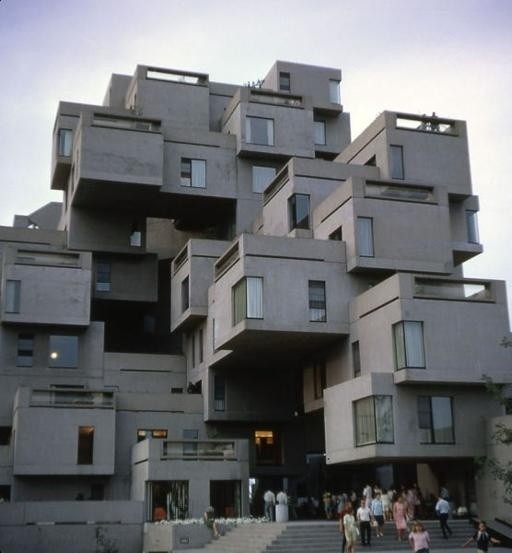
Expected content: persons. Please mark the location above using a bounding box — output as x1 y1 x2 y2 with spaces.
461 521 501 553
421 114 427 130
431 112 440 131
263 480 453 553
